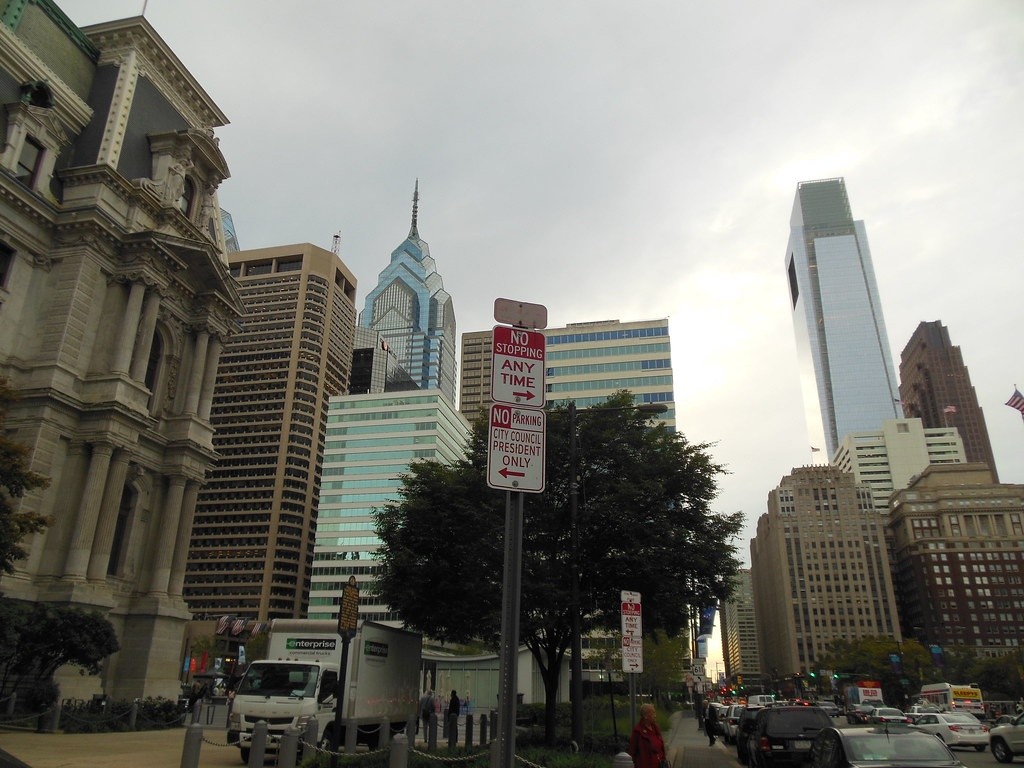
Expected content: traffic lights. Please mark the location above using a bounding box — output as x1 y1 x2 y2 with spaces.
740 685 743 689
732 691 735 695
810 667 815 677
833 671 838 679
771 693 775 698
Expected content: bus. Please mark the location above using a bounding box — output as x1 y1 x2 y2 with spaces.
921 683 986 719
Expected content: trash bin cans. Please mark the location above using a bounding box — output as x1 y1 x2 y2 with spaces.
90 693 108 712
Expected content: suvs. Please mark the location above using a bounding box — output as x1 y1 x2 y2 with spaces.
730 704 834 768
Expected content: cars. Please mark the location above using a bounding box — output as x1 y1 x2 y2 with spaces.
867 709 911 726
990 711 1024 762
844 703 876 724
806 726 970 768
914 714 992 751
710 702 745 740
816 701 840 717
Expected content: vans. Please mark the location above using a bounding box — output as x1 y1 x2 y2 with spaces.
748 695 775 706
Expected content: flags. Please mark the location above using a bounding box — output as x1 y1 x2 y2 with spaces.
231 618 248 636
1005 388 1024 420
251 623 267 640
216 615 236 635
895 399 904 405
811 447 820 452
944 404 957 414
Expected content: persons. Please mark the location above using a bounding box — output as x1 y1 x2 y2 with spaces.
446 690 460 744
702 700 719 746
419 689 435 743
630 703 666 768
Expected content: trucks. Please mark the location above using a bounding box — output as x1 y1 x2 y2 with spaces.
227 618 423 764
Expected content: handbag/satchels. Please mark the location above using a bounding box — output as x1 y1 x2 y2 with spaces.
422 708 434 722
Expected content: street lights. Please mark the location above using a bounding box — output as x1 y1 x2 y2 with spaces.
874 641 911 709
